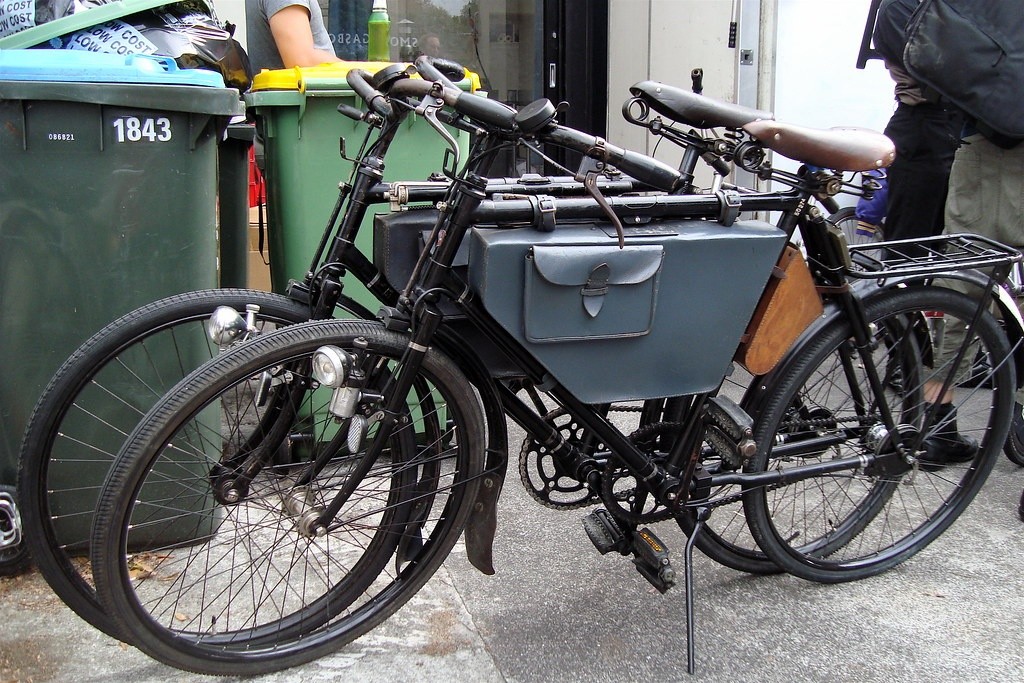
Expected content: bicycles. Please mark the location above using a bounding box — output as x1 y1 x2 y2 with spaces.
13 50 1024 678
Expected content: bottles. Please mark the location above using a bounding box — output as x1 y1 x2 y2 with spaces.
368 0 391 62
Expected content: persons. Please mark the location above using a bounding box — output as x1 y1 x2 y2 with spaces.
875 6 1024 471
416 32 442 58
244 0 346 181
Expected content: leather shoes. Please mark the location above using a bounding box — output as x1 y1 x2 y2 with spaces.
1002 414 1024 466
955 362 993 389
917 410 978 470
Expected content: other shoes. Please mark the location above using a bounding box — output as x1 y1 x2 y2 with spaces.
881 365 905 396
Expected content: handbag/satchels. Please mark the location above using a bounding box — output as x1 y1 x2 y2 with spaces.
902 0 1024 150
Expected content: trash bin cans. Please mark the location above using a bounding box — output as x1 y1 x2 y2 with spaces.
0 1 257 580
243 60 484 474
